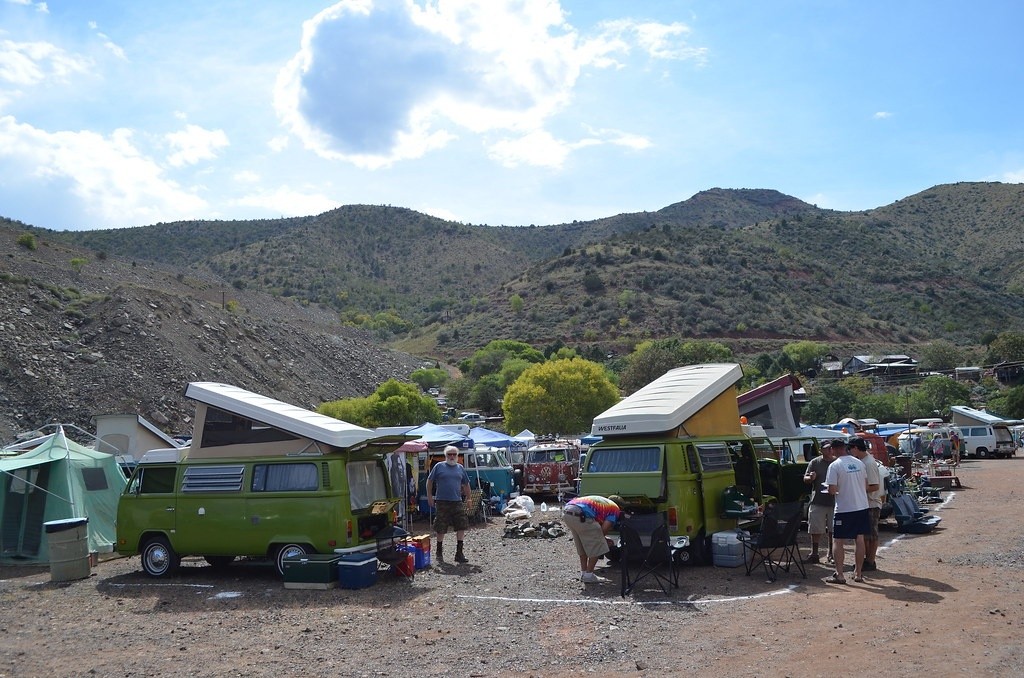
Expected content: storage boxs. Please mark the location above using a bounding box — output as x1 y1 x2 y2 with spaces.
712 530 752 567
338 553 378 589
283 554 343 590
399 544 431 570
930 478 952 490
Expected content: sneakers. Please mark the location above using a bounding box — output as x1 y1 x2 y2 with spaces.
580 572 607 584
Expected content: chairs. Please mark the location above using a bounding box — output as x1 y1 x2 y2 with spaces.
617 511 681 599
737 499 808 584
891 496 938 533
899 494 942 527
374 534 415 582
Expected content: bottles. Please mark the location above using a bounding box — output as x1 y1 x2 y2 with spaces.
540 502 547 512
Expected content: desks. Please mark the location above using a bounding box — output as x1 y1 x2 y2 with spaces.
931 463 956 476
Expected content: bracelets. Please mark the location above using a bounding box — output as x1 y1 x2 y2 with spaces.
467 500 472 502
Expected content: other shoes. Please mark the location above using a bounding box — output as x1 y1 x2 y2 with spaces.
826 573 846 584
455 551 468 562
851 572 863 582
436 553 444 566
853 560 876 571
799 554 819 564
825 554 835 564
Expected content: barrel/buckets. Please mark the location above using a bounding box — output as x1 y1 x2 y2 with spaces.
43 518 91 581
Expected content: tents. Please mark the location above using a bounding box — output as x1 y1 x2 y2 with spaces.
0 424 137 569
397 421 488 525
467 427 529 497
513 429 536 447
577 434 603 494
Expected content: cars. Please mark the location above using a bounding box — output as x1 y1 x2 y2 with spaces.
437 399 446 406
427 388 439 397
462 413 481 421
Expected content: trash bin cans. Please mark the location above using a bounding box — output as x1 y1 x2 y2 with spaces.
45 518 91 581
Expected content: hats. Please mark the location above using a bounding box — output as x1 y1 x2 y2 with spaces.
845 437 864 446
950 431 954 433
829 438 844 449
934 433 940 437
608 495 626 510
821 439 832 449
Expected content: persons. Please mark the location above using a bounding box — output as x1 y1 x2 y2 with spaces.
800 440 837 564
949 430 961 467
390 454 404 496
824 438 868 584
426 445 472 563
564 494 625 583
912 432 923 459
581 453 597 472
847 435 882 571
885 433 902 460
926 433 946 460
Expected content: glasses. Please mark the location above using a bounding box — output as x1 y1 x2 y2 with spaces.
446 453 457 456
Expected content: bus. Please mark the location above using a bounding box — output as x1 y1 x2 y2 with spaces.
522 442 581 500
112 381 424 579
897 427 966 461
580 362 820 560
948 406 1024 459
419 448 515 513
735 374 892 521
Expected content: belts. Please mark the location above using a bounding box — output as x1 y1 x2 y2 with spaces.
563 511 580 517
